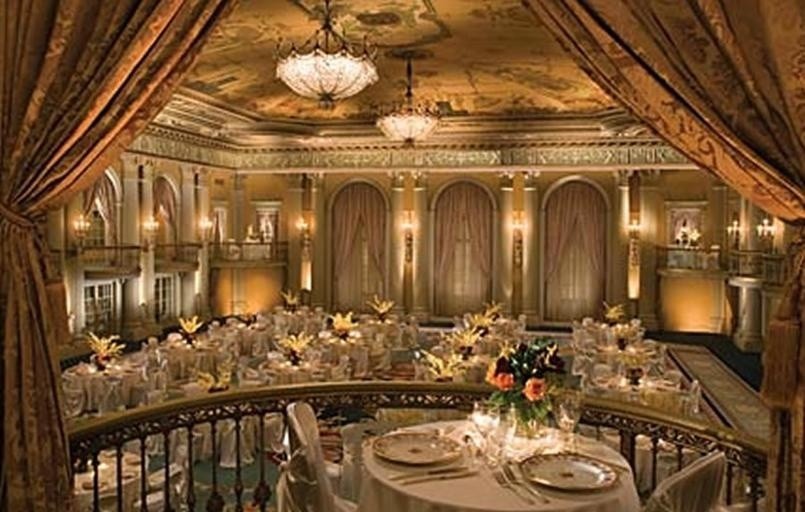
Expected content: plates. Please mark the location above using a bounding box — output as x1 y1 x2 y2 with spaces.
520 453 618 493
374 432 465 464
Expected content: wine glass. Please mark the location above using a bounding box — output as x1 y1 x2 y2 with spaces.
472 395 583 459
574 326 701 394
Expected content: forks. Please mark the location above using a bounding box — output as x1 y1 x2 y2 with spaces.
492 463 555 506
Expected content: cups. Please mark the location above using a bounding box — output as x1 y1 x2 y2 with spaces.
441 306 505 376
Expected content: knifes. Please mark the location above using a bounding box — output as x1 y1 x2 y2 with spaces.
386 466 481 485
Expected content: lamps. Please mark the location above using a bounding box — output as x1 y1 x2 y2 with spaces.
270 0 389 112
372 44 451 149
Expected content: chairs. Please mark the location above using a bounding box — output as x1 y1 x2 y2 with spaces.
62 290 727 512
